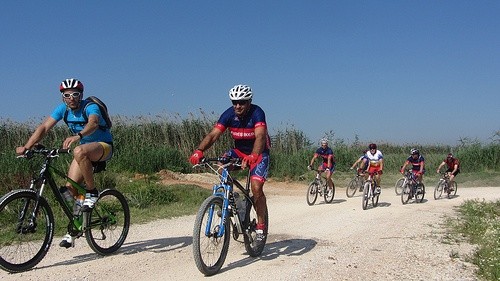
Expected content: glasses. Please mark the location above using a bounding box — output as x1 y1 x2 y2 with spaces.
232 100 249 105
370 148 376 150
62 92 82 98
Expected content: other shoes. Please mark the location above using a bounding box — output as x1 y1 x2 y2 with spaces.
376 186 381 194
416 186 422 193
317 186 320 191
449 185 455 191
359 185 364 192
328 188 332 196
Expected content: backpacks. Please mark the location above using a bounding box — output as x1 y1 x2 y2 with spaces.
63 96 112 132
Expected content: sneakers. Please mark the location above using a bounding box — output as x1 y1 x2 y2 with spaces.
255 229 267 243
59 230 83 248
81 190 100 212
216 205 233 217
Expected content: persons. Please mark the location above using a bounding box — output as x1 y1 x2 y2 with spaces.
400 148 428 198
307 138 335 196
349 143 383 195
188 85 272 243
435 153 459 197
15 79 116 248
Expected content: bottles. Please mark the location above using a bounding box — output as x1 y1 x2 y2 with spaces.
74 193 85 216
234 192 244 209
60 186 74 205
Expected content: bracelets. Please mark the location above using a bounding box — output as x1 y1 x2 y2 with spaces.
76 132 82 139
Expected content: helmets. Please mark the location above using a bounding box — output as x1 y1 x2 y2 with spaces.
447 154 453 158
228 85 253 100
59 78 83 93
363 150 367 152
409 148 416 153
369 144 377 148
321 138 328 145
411 150 419 155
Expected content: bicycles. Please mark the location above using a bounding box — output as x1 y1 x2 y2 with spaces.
433 171 458 200
361 169 382 210
394 165 411 196
0 143 131 275
190 151 269 278
400 170 427 205
346 168 368 198
306 164 336 206
